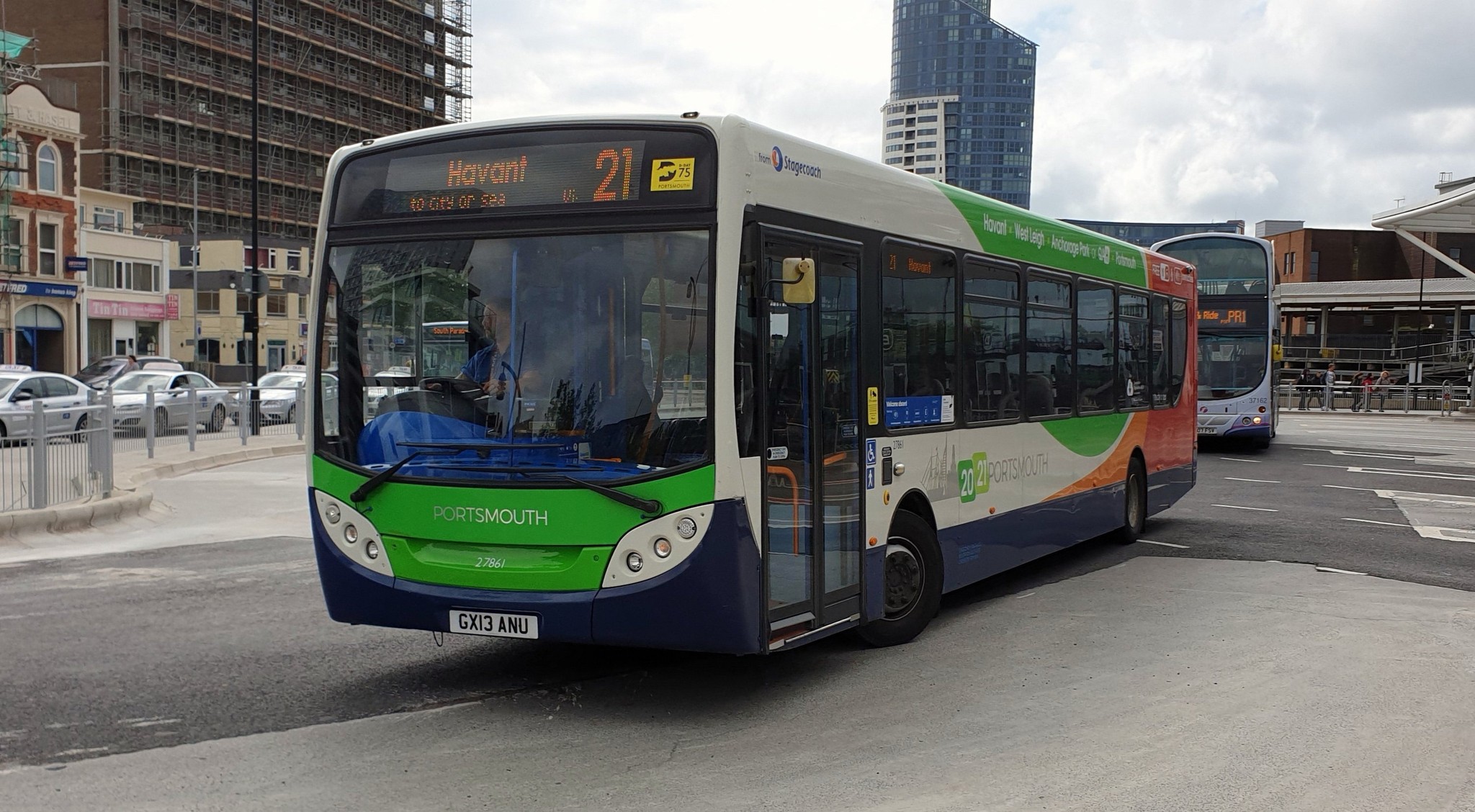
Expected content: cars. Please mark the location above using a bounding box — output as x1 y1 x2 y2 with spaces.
367 365 412 417
0 363 104 450
228 363 340 426
103 367 232 439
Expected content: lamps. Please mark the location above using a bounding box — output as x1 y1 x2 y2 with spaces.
181 342 184 347
231 343 234 349
261 343 264 349
223 342 226 348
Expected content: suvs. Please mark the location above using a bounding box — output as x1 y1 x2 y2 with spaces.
67 353 185 393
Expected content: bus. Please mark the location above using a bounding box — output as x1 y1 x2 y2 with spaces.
1146 230 1285 452
421 320 655 417
299 107 1200 660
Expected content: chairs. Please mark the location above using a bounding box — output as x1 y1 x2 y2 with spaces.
839 335 1139 415
1225 278 1266 295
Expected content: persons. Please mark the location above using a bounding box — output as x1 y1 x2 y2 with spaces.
122 355 139 375
1296 363 1335 411
455 297 547 396
1351 371 1390 412
296 356 305 365
